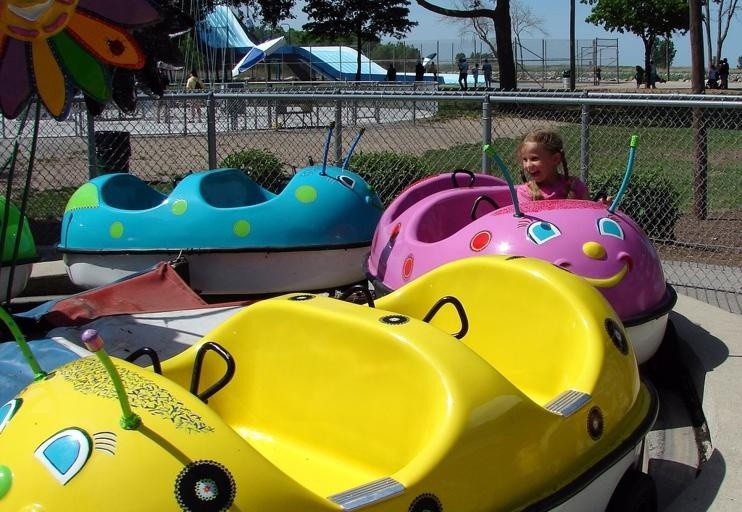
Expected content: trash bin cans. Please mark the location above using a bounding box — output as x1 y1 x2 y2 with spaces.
562 70 570 88
94 131 130 178
226 84 245 114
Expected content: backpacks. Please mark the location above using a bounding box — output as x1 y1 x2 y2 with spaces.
190 77 202 92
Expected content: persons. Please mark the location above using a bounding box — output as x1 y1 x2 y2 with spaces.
708 65 720 88
187 69 204 124
635 66 643 89
482 60 492 87
157 72 170 124
385 64 399 82
459 59 468 91
416 62 426 83
722 58 729 89
596 68 601 85
473 63 479 87
719 61 724 88
648 60 657 88
430 61 437 74
516 130 613 204
